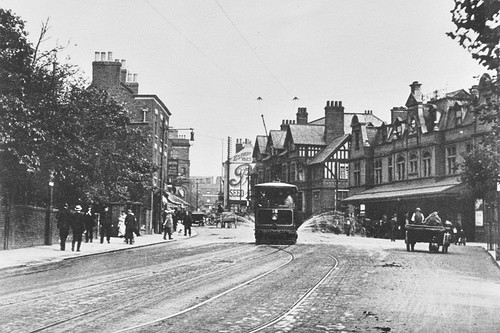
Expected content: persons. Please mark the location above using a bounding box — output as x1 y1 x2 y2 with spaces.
100 206 112 244
118 209 141 244
72 205 83 251
84 205 95 243
163 203 225 241
335 205 466 248
56 203 71 250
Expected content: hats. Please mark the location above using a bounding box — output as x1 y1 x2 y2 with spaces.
416 207 421 210
74 204 82 210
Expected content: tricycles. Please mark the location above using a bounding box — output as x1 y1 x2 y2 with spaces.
404 223 451 253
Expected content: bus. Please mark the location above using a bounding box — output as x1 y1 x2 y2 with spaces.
254 181 298 245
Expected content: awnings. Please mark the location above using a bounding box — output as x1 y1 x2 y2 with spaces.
342 175 470 203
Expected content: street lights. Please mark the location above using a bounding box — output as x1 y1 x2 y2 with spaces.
159 117 195 233
